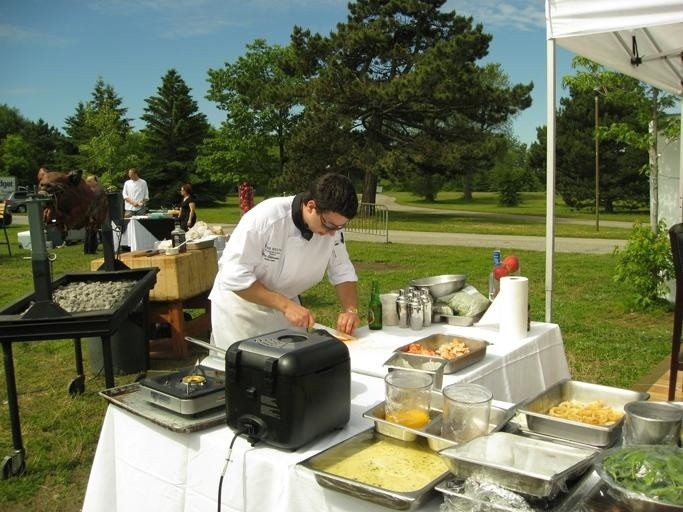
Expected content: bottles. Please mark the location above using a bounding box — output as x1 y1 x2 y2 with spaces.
367 278 432 331
487 249 506 302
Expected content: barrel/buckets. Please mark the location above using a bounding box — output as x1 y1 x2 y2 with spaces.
91 308 147 379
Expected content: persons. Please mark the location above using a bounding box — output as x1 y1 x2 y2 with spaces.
208 171 360 357
181 185 196 231
84 174 99 255
122 168 150 228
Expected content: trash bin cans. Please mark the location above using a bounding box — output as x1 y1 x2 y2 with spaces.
87 290 150 377
45 222 65 247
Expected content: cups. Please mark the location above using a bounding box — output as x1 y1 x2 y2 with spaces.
440 380 493 441
383 370 432 428
623 402 683 446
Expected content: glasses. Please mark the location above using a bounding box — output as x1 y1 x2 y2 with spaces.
319 211 343 231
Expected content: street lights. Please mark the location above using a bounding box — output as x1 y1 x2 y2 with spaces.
591 86 604 233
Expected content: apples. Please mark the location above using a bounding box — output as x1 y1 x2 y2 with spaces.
502 256 520 273
493 265 508 280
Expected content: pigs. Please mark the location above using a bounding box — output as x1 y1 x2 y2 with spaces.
37 167 108 230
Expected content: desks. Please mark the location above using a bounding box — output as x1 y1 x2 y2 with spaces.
91 245 216 359
78 371 513 511
334 320 572 403
105 208 191 252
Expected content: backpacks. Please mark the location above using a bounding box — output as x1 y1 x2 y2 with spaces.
186 210 197 229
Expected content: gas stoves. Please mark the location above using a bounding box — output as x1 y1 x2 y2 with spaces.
138 364 225 418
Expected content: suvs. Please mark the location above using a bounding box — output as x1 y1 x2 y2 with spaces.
0 188 28 214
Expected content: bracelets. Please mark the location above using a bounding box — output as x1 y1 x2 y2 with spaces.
344 307 359 313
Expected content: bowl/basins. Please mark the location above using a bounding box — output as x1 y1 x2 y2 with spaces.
592 443 683 512
409 274 466 298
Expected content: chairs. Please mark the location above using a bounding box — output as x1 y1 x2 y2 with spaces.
662 222 683 403
